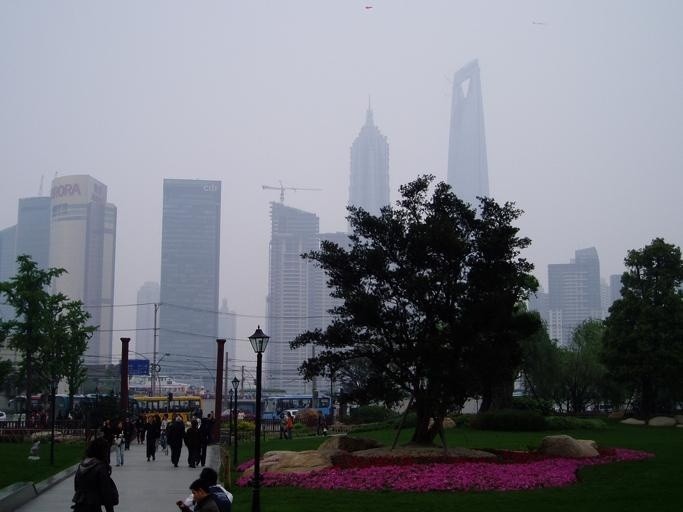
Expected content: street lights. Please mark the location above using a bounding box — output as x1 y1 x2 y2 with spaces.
228 325 271 512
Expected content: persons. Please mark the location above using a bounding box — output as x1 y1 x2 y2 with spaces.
237 408 245 420
26 403 83 429
317 410 328 437
184 468 233 512
279 413 288 440
97 410 215 468
70 440 118 512
286 411 293 440
176 479 220 512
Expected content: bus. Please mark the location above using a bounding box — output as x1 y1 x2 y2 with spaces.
222 393 333 425
7 393 203 428
